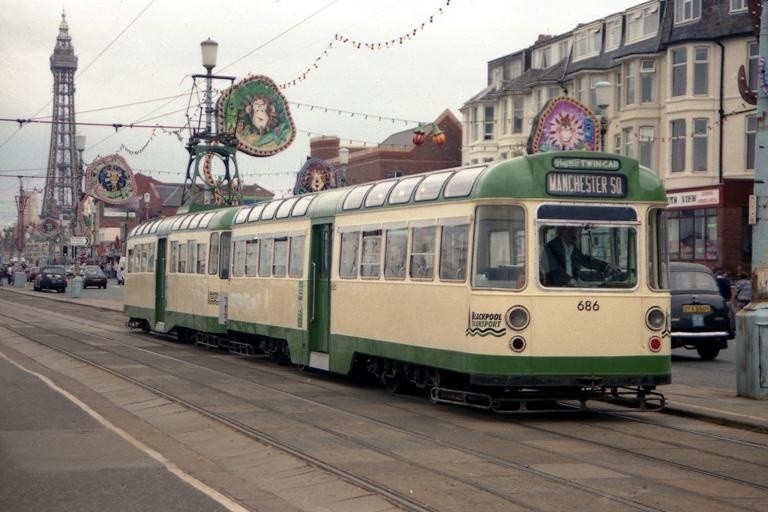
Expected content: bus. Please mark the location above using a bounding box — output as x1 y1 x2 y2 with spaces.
122 150 672 416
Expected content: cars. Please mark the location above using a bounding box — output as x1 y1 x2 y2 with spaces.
24 265 68 293
79 265 107 289
669 261 736 361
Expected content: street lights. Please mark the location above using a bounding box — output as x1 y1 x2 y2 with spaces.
200 36 218 205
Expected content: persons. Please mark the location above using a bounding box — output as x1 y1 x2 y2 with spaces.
716 271 735 339
5 262 17 286
679 239 693 258
735 273 753 308
21 261 27 269
99 252 125 285
541 223 621 287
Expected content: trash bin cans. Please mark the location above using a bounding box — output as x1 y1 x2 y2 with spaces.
14 272 26 288
66 276 82 298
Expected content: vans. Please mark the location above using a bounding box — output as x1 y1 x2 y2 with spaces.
117 257 125 285
